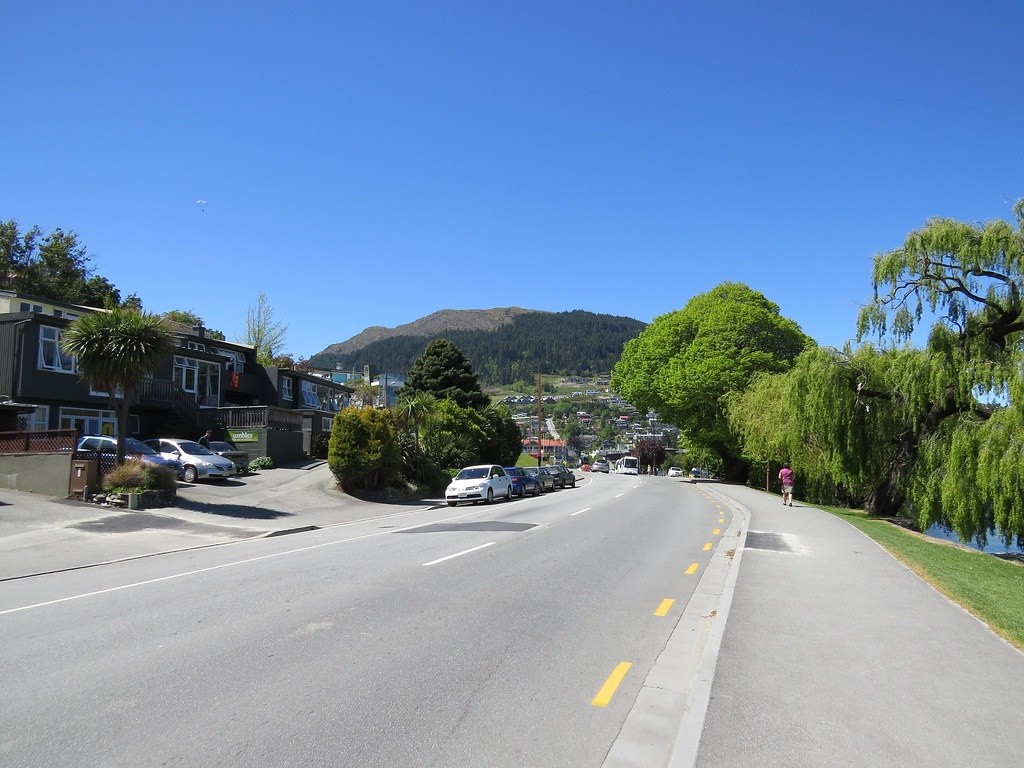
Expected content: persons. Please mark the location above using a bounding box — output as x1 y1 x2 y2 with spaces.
778 462 795 507
647 464 652 476
198 430 213 450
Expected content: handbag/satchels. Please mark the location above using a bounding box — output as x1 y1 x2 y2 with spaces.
779 479 783 485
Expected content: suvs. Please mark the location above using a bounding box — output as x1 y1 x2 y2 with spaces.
77 434 184 478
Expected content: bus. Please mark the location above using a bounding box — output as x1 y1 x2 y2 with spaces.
612 457 639 475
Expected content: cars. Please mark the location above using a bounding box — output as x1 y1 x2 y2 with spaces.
502 468 542 498
524 467 556 493
590 459 609 474
205 440 251 469
546 465 576 489
142 439 237 484
668 467 683 477
445 465 514 506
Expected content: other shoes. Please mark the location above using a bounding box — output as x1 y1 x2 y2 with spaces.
788 503 792 506
783 503 786 505
